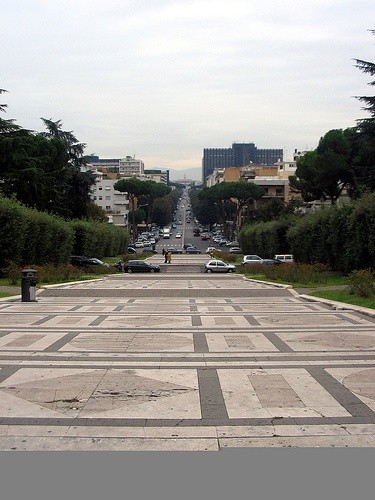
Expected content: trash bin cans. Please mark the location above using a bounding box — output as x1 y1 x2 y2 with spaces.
21 268 38 302
153 245 156 250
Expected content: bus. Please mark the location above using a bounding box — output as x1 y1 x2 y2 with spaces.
274 254 294 263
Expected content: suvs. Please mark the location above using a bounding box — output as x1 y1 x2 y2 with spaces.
205 247 222 254
184 246 201 255
79 258 109 268
123 260 160 273
143 247 157 255
70 256 87 266
240 255 267 265
127 247 137 254
183 243 195 249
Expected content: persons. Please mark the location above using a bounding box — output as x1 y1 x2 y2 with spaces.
166 251 171 263
164 250 168 263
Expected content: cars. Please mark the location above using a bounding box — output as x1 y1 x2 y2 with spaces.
162 247 183 254
128 194 239 248
262 258 281 265
228 247 241 254
201 259 236 274
